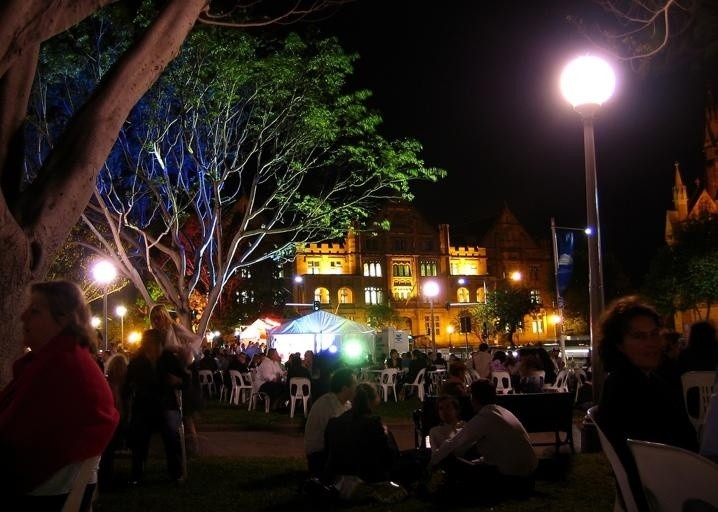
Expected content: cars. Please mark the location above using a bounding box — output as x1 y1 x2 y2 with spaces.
422 341 556 359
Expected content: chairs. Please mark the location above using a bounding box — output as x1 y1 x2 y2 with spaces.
680 370 718 438
623 436 718 510
175 346 595 422
60 453 103 511
584 405 639 509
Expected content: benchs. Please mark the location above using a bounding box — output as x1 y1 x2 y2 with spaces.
410 392 578 462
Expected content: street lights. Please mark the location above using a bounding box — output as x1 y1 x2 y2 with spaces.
421 277 441 361
546 45 626 355
115 304 127 349
293 274 303 314
446 326 455 346
92 257 119 353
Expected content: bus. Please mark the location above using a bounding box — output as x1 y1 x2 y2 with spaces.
552 335 590 361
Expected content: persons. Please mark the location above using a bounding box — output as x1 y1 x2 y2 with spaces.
188 341 557 512
1 280 200 512
235 336 237 341
593 295 718 512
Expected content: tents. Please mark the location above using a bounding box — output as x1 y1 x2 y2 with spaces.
266 310 377 361
238 319 275 344
